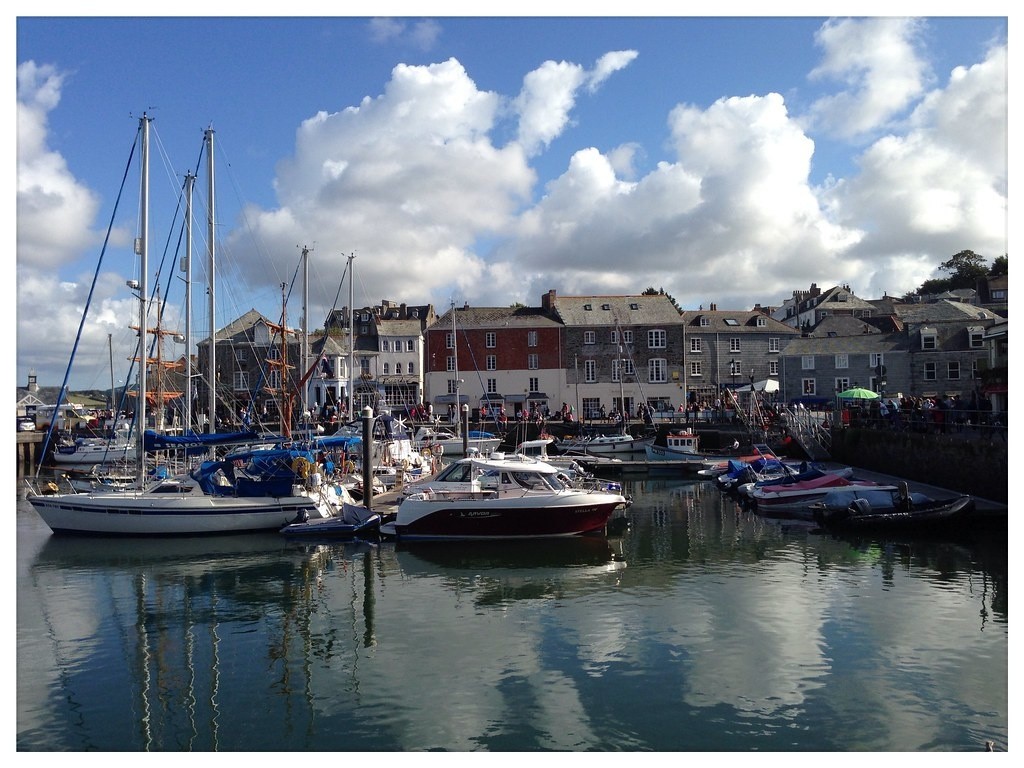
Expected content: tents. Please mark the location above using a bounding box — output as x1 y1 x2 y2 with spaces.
735 379 780 393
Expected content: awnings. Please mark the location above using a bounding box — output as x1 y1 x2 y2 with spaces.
791 395 835 404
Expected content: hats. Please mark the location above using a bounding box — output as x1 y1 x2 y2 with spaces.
350 455 358 459
218 470 225 476
333 468 341 473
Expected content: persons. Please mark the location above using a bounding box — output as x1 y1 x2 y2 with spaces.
427 443 443 464
318 451 329 475
174 410 179 426
219 397 348 431
846 394 996 435
410 401 575 422
742 402 804 443
727 438 739 455
599 392 738 424
86 408 134 421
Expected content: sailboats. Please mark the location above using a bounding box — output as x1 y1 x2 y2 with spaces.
25 98 975 544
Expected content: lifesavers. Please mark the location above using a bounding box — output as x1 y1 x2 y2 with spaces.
400 459 409 470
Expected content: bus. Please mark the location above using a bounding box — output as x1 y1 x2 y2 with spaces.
36 403 98 433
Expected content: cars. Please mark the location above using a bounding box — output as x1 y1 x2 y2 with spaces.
18 417 36 433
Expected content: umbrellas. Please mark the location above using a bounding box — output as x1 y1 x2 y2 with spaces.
837 388 879 407
